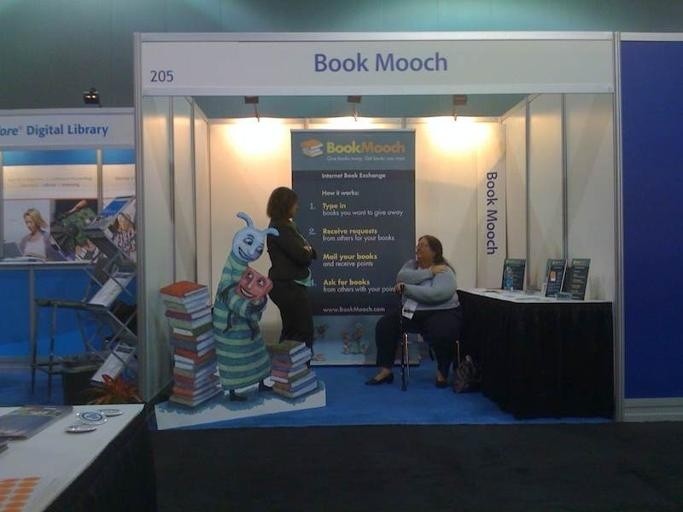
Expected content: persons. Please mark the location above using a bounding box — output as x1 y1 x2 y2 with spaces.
18 207 49 260
265 186 317 370
363 234 464 389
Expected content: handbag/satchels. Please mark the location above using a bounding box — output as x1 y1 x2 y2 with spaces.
451 352 481 393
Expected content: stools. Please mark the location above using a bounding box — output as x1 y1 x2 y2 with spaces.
401 331 461 393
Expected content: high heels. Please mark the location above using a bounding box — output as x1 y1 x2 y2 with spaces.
434 372 450 389
365 372 395 386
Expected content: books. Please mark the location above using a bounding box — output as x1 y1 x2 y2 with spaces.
159 281 224 408
269 339 318 399
0 404 73 440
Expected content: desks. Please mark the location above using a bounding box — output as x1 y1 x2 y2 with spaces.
457 287 615 419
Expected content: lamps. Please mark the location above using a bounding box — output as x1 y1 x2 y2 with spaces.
83 87 103 107
